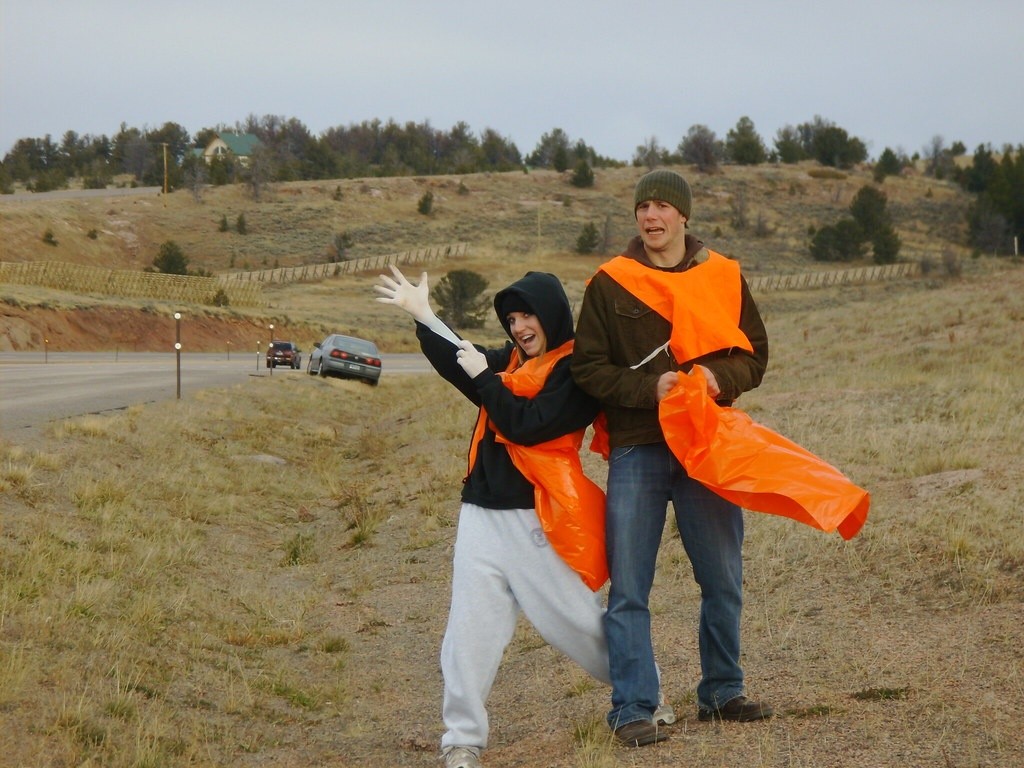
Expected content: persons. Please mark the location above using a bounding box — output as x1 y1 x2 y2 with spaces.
571 171 777 748
370 260 677 767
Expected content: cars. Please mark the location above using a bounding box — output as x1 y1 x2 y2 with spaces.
306 333 382 386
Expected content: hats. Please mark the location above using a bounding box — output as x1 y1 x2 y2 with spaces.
634 169 692 221
500 288 536 321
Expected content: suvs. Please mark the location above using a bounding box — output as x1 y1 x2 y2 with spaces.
266 340 302 369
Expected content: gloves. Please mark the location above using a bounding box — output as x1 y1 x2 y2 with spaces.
373 265 461 349
456 340 488 379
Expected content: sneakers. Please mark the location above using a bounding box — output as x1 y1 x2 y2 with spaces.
614 720 669 747
698 691 772 722
438 742 481 768
651 689 675 726
